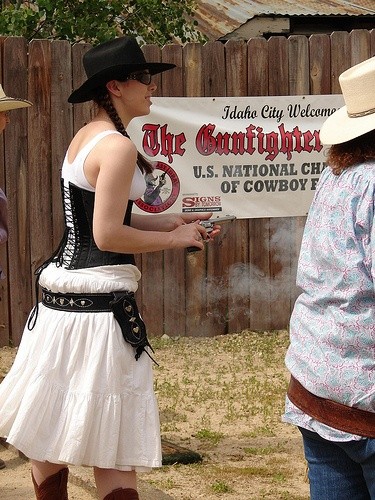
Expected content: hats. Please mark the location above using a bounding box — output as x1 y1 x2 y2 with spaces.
319 56 375 144
67 37 177 103
0 83 34 113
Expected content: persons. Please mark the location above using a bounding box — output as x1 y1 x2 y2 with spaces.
0 84 33 281
282 55 375 500
0 35 220 500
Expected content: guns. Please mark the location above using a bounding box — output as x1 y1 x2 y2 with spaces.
184 215 236 255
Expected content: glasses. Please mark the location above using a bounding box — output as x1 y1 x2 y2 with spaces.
117 73 152 85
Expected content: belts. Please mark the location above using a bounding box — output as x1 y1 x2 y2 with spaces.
287 373 375 439
